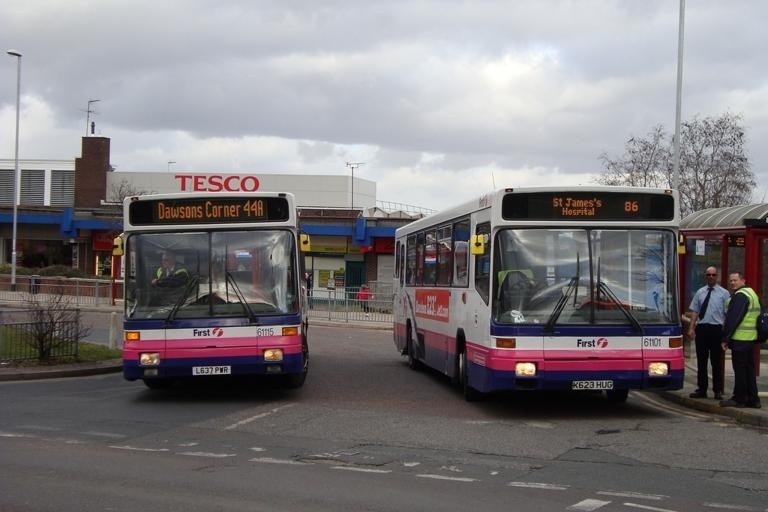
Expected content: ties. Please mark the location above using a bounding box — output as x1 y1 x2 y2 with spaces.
698 287 714 320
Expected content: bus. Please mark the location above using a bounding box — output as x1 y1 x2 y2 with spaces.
393 187 686 405
112 192 310 390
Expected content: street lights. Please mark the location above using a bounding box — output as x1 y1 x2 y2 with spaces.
6 49 22 291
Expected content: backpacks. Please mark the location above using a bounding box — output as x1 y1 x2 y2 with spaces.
755 311 768 342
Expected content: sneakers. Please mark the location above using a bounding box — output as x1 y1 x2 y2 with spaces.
719 393 761 408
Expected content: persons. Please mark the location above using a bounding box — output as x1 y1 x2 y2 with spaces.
151 252 189 306
687 265 762 408
355 283 374 314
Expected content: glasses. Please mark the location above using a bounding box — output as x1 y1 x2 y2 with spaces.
705 273 718 277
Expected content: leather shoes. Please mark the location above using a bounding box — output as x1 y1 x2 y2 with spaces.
713 390 724 399
689 387 707 398
